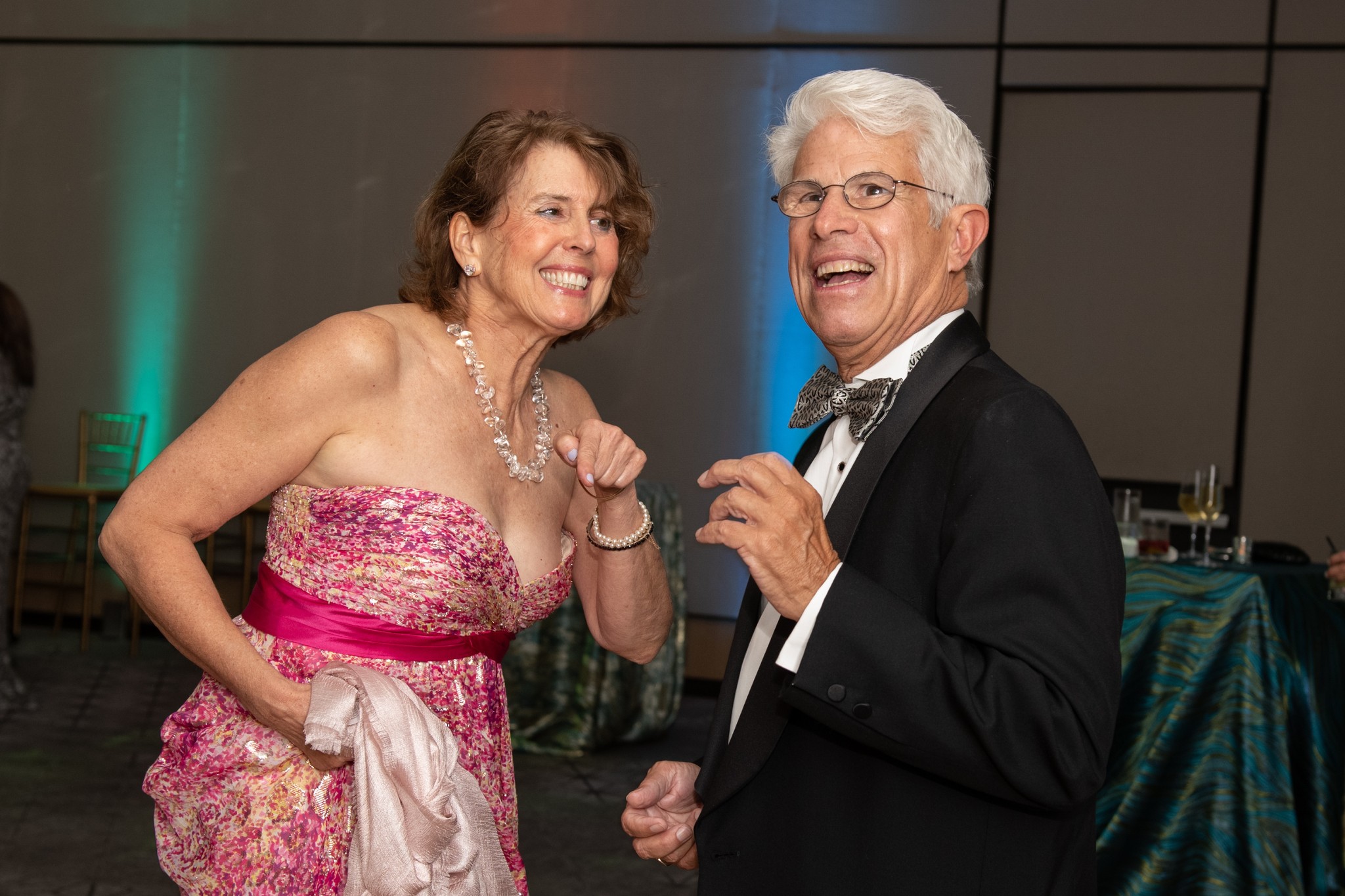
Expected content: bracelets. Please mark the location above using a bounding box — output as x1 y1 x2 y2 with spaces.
588 498 660 550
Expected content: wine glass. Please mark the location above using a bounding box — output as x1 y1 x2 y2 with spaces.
1174 465 1224 567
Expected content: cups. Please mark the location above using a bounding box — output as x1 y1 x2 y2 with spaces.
1111 487 1167 561
1232 536 1252 564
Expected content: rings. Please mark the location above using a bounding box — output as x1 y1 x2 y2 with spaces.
657 858 670 867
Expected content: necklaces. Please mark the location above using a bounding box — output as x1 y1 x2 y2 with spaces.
442 310 554 485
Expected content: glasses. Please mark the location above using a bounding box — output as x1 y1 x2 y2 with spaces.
771 173 955 217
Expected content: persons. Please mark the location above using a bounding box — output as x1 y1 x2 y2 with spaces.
620 69 1128 895
97 107 676 896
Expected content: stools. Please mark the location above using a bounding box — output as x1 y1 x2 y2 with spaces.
12 411 273 657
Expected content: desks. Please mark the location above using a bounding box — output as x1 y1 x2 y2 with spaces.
498 486 688 759
1093 558 1344 896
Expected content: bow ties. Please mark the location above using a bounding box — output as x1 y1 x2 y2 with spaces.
790 364 903 441
